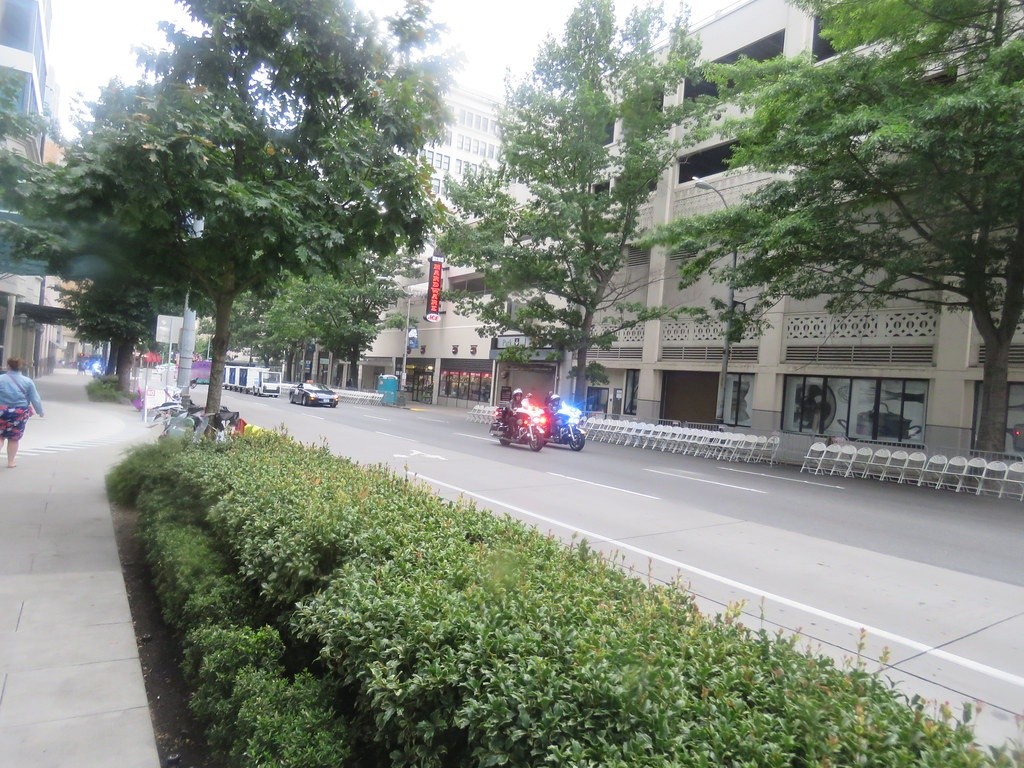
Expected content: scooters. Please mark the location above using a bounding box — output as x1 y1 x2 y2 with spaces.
148 376 263 445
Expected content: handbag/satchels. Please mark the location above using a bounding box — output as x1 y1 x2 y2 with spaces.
28 405 33 417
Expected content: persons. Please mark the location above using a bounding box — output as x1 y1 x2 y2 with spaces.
77 362 86 374
0 356 45 468
548 394 562 435
504 387 524 436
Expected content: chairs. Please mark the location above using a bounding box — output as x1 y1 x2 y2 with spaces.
467 404 500 424
800 444 1024 501
579 416 782 468
331 388 384 406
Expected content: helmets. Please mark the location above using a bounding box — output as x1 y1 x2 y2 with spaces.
550 394 560 404
512 388 522 399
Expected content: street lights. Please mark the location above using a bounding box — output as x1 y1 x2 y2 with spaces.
385 276 414 406
693 177 740 434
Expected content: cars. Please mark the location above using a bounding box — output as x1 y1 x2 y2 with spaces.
289 379 339 408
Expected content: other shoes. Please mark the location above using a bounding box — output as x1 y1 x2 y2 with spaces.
8 464 16 468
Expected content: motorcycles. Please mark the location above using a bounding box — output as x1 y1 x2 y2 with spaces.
487 392 547 452
540 399 589 451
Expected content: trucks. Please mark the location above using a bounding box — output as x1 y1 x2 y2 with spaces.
223 365 282 397
